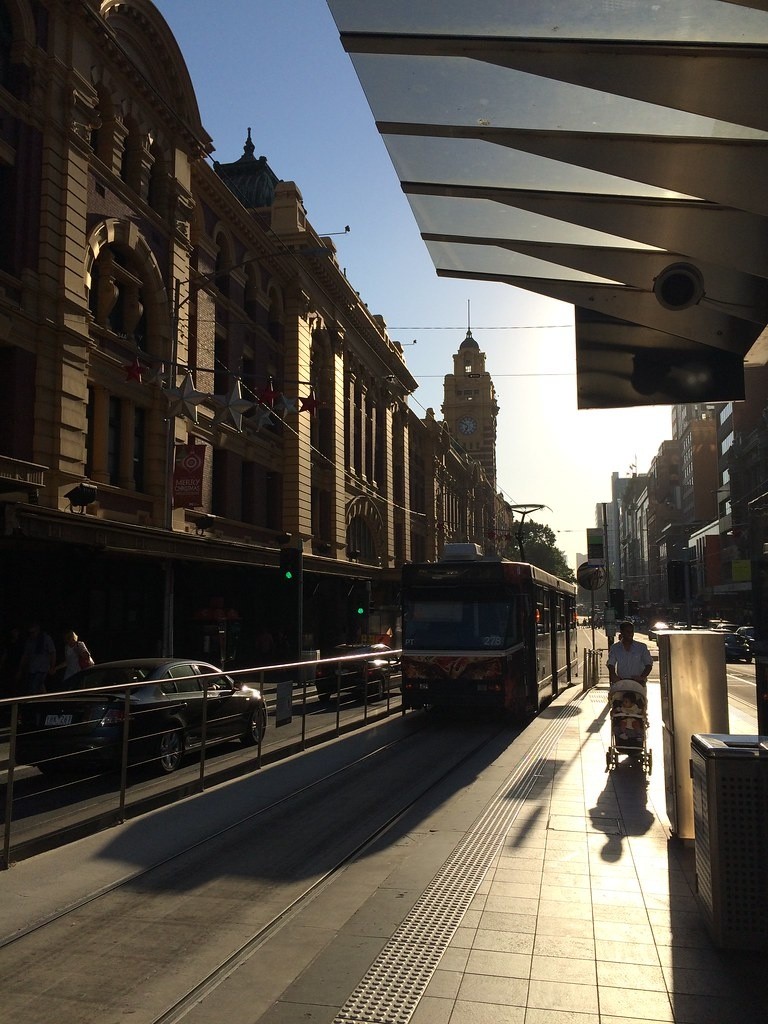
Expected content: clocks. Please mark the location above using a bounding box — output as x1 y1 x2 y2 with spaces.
458 417 477 435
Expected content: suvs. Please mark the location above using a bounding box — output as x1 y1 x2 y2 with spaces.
314 643 401 704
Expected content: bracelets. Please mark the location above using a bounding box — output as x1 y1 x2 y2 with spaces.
609 673 616 675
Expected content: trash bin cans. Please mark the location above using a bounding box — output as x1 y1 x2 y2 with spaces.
692 732 768 956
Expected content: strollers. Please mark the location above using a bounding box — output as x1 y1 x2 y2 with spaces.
606 678 652 775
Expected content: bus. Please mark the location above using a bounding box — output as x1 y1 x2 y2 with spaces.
401 504 579 720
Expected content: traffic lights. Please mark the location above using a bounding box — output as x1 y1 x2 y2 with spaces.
280 548 299 587
354 589 369 619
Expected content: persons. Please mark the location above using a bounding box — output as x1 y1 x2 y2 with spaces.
1 625 25 691
16 624 56 693
606 621 653 757
610 693 642 741
55 629 90 679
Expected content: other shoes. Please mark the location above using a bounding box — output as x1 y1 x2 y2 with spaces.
636 734 643 742
619 733 628 739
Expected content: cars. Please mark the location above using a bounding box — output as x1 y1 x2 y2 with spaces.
14 658 269 785
648 619 755 664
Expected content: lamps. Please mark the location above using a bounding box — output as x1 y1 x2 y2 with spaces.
317 225 350 236
195 514 216 537
274 532 293 547
400 339 417 347
316 543 332 555
346 549 361 562
63 483 98 514
